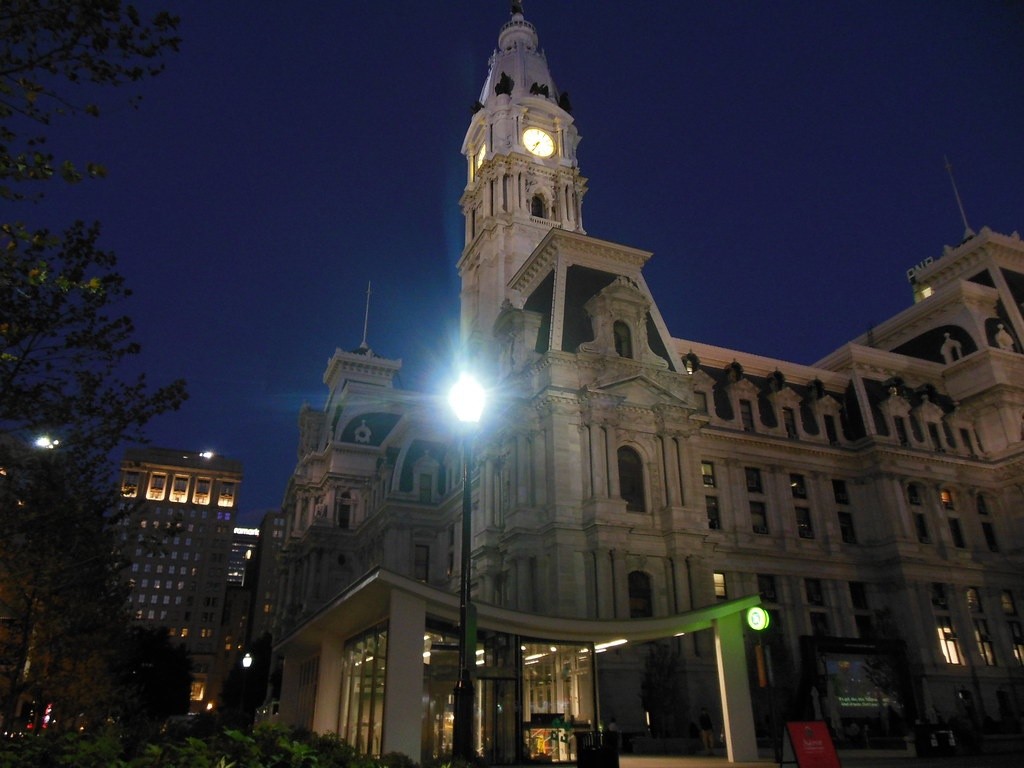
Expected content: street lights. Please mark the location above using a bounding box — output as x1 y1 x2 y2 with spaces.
424 340 506 768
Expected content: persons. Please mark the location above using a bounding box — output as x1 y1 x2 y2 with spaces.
698 707 718 757
608 717 621 754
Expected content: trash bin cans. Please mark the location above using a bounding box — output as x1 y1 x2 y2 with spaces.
574 731 619 768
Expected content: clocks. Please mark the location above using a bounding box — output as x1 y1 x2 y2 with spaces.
524 127 556 159
478 140 485 170
